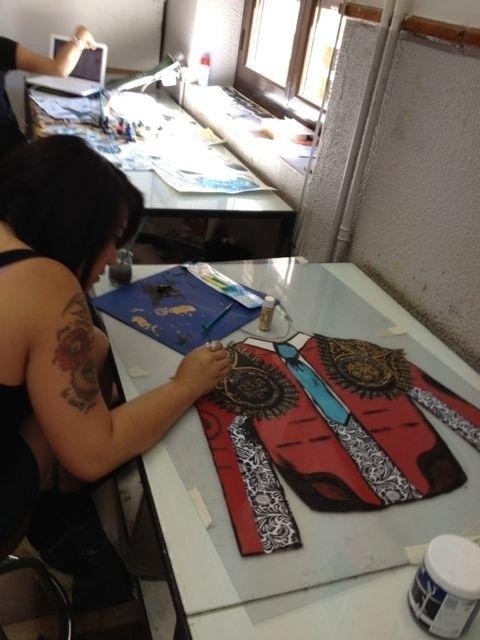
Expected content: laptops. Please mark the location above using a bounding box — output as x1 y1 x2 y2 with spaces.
23 32 109 98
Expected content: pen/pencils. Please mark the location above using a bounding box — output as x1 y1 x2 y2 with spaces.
200 302 234 333
99 87 104 118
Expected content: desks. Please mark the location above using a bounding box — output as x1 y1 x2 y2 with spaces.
17 66 299 265
82 245 478 639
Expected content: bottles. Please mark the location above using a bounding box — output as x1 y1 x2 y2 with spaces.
98 116 145 142
258 295 275 330
404 533 480 640
198 53 211 88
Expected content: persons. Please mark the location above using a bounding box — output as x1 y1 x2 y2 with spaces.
0 131 237 602
1 25 98 210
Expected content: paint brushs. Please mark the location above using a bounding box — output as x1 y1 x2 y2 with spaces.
120 213 149 265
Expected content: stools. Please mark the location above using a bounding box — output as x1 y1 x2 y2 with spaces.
0 553 77 640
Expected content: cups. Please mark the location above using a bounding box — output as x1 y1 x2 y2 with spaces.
108 249 134 289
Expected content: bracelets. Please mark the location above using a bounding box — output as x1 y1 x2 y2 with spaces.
70 35 86 51
